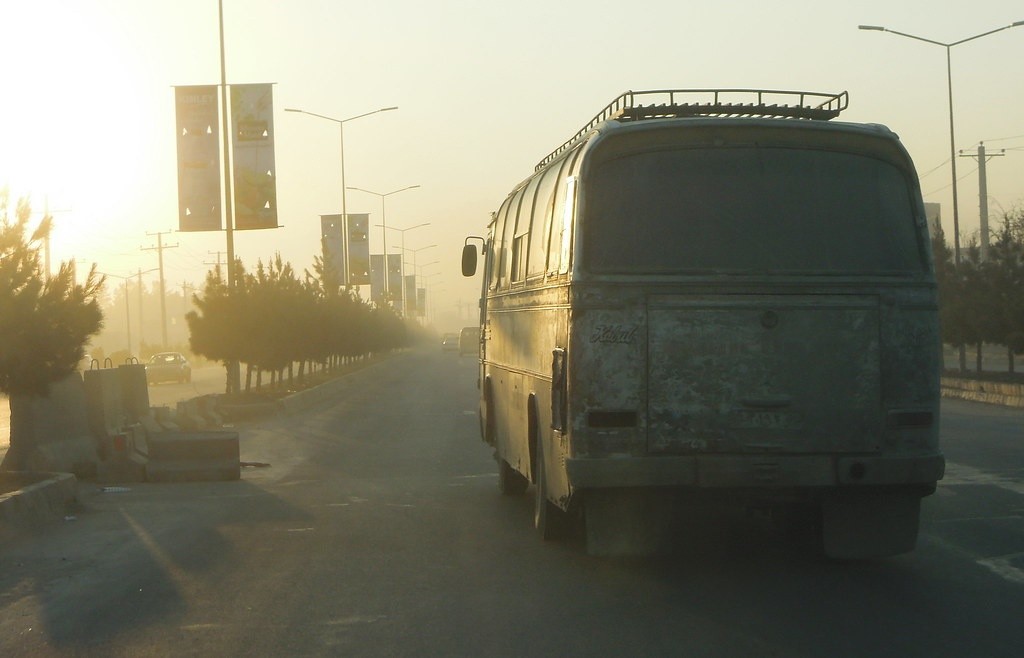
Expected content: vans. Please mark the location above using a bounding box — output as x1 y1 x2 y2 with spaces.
458 326 481 356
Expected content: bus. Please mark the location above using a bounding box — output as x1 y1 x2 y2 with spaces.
458 82 945 553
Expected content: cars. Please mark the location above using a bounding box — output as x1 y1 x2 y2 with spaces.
145 352 193 384
444 337 458 351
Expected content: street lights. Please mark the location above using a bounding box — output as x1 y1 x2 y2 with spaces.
283 105 398 291
860 21 1024 263
95 266 159 361
374 223 431 319
344 185 421 300
403 261 439 313
